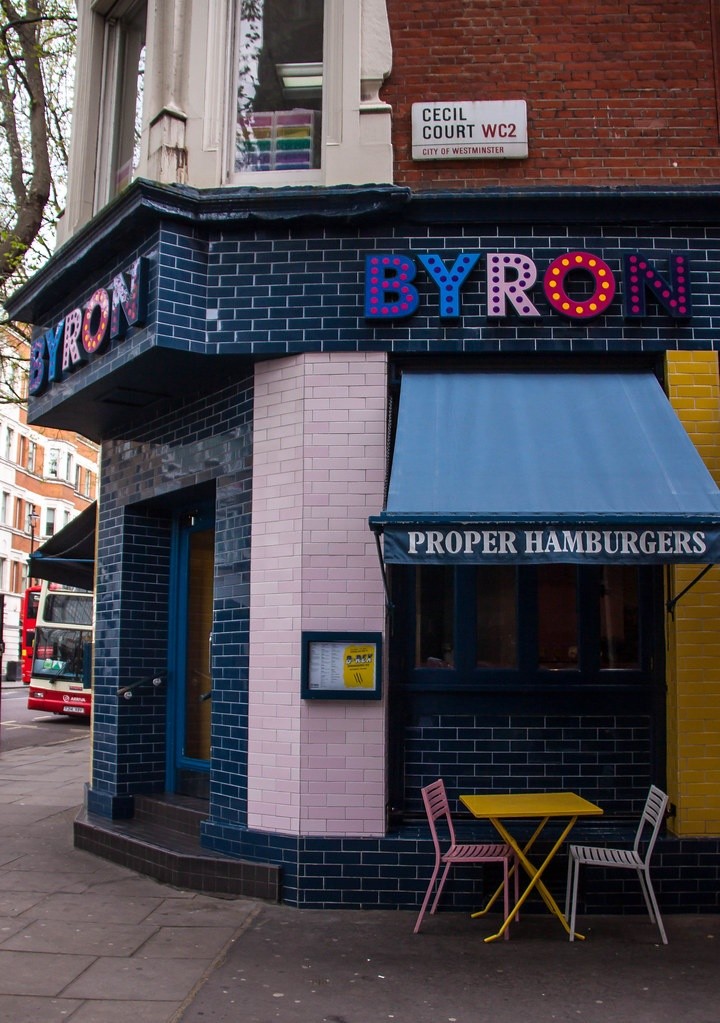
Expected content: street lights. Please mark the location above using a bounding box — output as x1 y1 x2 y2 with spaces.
28 509 40 587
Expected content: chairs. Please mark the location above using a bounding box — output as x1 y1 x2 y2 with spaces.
413 778 520 940
564 784 669 945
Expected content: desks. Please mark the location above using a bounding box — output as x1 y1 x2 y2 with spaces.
460 792 603 944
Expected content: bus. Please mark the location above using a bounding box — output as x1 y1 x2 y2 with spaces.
28 578 95 720
18 587 69 687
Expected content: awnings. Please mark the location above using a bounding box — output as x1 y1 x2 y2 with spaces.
368 372 719 610
28 499 97 592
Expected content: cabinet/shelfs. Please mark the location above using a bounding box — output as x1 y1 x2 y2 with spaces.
235 109 321 171
